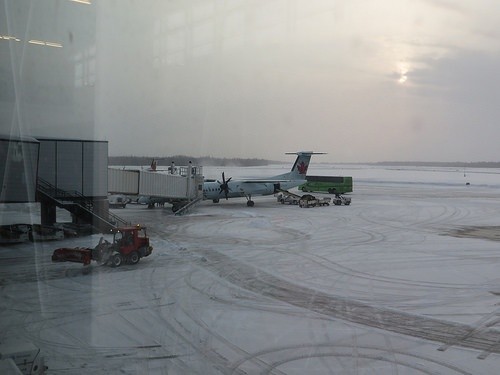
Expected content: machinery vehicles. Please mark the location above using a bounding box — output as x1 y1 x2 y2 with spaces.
53 224 152 267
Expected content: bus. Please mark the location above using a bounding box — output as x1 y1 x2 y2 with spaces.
300 173 354 194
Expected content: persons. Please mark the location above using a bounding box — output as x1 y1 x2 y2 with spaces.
123 232 131 244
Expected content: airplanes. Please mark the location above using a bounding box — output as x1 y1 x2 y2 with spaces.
201 149 327 208
